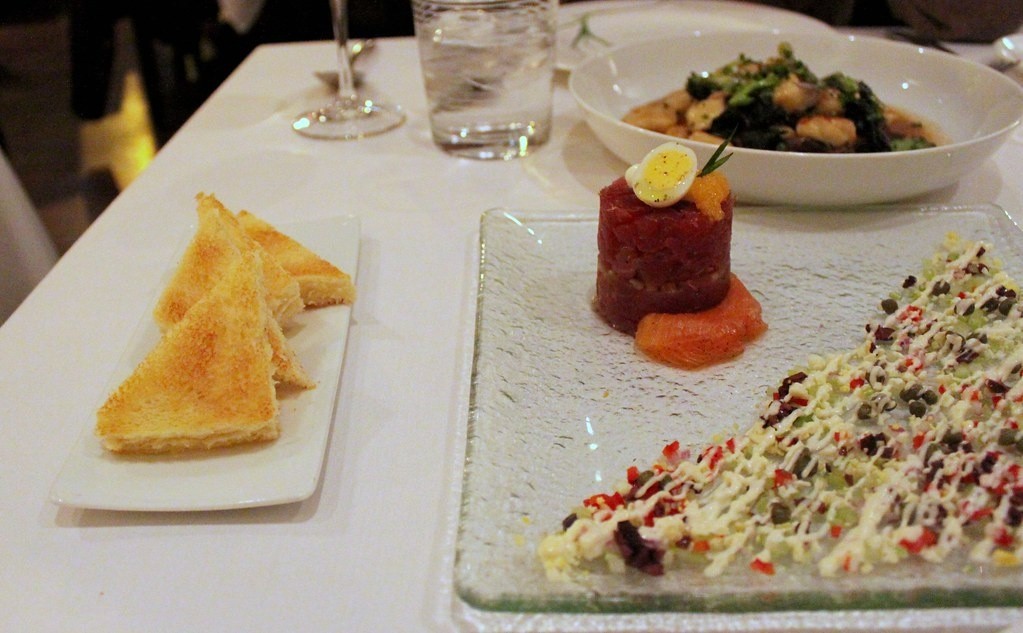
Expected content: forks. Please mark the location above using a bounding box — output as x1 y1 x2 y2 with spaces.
888 29 1020 74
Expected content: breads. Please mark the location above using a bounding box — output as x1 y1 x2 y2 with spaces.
90 193 355 456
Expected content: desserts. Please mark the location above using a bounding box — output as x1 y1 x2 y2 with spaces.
589 121 738 337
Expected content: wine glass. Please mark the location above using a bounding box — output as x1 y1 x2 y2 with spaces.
291 1 406 139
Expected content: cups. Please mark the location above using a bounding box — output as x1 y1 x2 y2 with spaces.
410 0 553 163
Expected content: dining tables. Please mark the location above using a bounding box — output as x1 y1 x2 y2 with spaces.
0 37 1022 633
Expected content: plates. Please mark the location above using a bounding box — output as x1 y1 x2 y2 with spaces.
567 29 1023 205
449 209 1023 618
48 231 363 510
548 0 839 73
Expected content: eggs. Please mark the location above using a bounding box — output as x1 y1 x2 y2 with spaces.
631 141 696 207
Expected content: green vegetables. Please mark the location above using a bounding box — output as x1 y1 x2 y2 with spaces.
685 40 936 152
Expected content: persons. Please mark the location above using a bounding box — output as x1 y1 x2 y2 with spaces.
752 0 1023 47
135 0 416 154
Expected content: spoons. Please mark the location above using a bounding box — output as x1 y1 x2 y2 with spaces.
315 36 377 87
